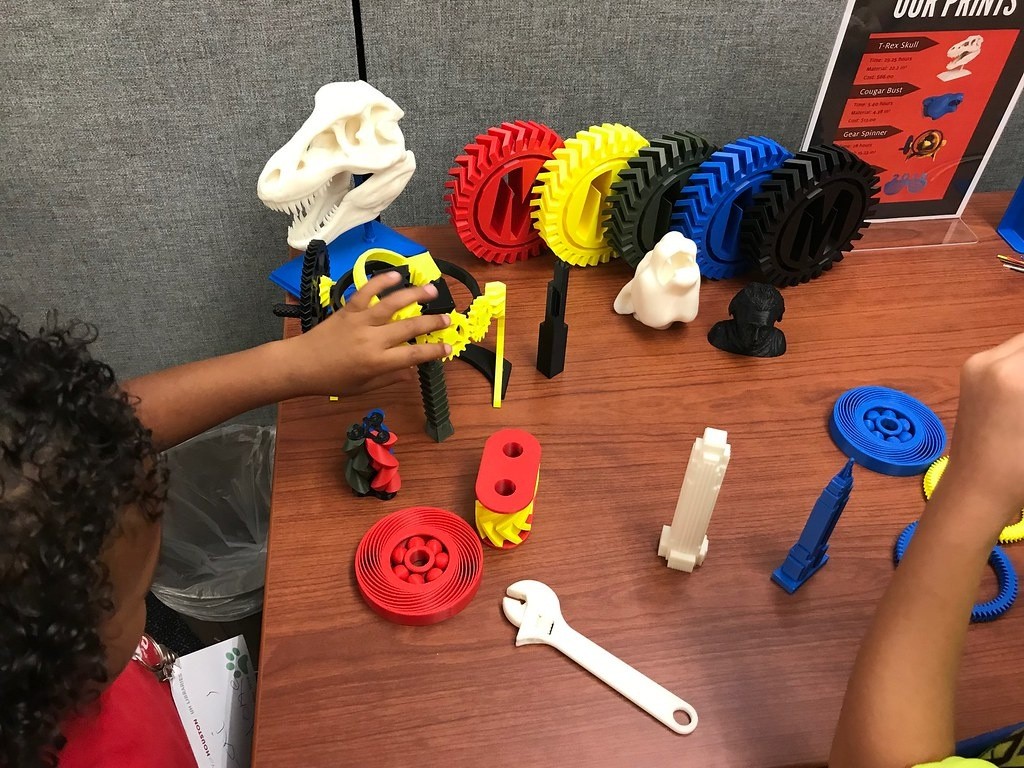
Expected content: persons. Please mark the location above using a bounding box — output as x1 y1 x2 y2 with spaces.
827 332 1024 768
1 270 452 768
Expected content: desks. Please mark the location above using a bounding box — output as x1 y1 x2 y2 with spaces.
251 192 1024 768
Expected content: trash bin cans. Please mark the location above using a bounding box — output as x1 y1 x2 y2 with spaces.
147 423 277 672
